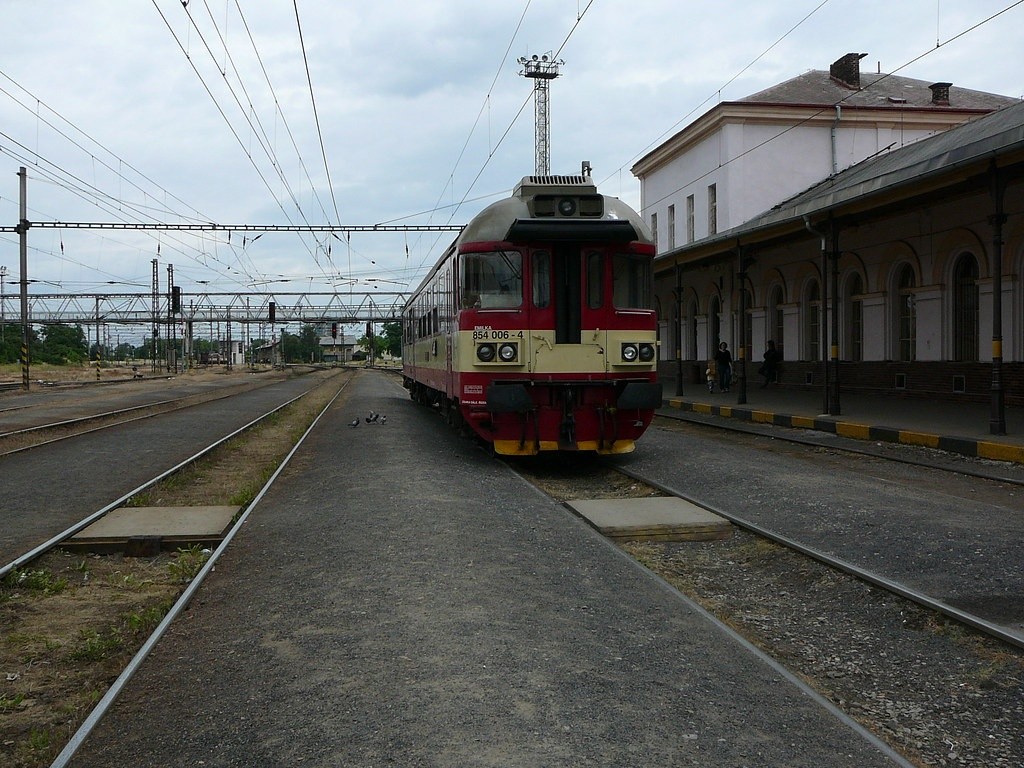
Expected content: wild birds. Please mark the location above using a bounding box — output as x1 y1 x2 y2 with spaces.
347 410 387 428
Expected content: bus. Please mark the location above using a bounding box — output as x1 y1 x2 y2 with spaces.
402 160 663 474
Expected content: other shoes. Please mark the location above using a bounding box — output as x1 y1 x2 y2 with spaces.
721 389 725 393
724 388 729 392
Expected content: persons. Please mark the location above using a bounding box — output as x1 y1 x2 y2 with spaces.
758 339 777 386
706 342 734 393
